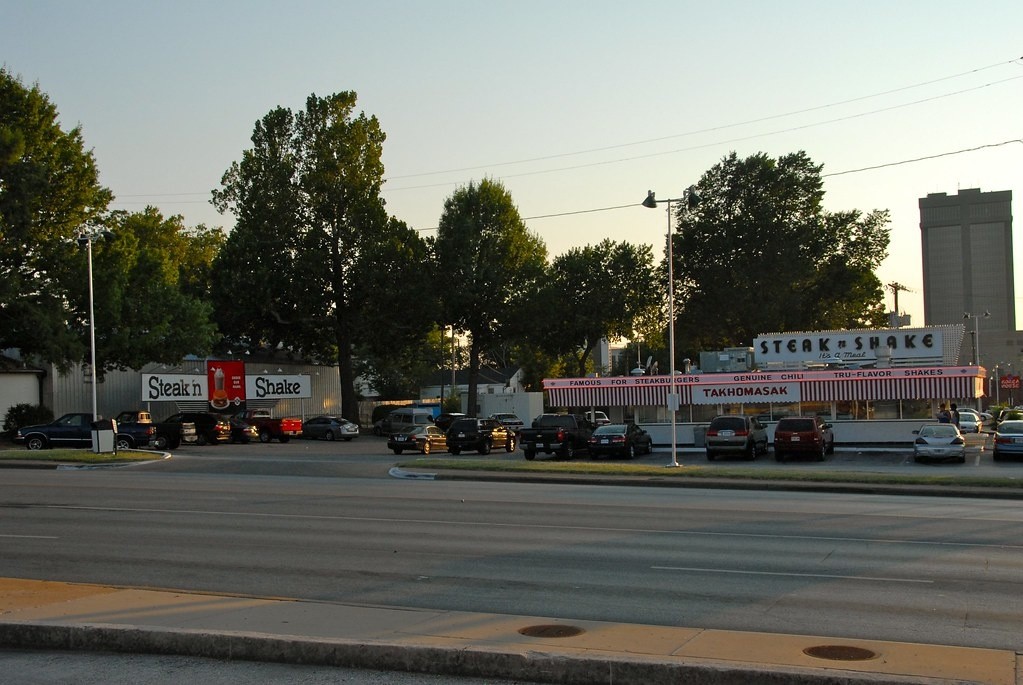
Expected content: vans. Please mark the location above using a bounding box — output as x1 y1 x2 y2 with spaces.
373 407 431 436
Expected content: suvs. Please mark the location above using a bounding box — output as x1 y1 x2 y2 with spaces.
704 413 768 461
446 418 516 456
772 416 835 463
160 411 231 446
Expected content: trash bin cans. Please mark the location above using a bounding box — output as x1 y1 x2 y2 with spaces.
91 419 116 454
693 427 706 447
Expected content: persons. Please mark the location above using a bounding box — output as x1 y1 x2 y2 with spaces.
935 403 951 423
948 403 960 428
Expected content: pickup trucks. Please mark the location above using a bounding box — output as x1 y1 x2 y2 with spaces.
230 409 302 444
114 410 198 450
516 412 592 460
15 413 157 450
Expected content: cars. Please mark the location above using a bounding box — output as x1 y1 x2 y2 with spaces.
586 422 652 461
488 412 524 431
427 413 470 433
388 424 448 454
228 417 259 441
303 416 360 441
912 406 1022 464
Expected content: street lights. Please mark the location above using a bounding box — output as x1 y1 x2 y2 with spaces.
78 204 103 432
990 361 1014 406
962 307 992 364
639 185 702 470
